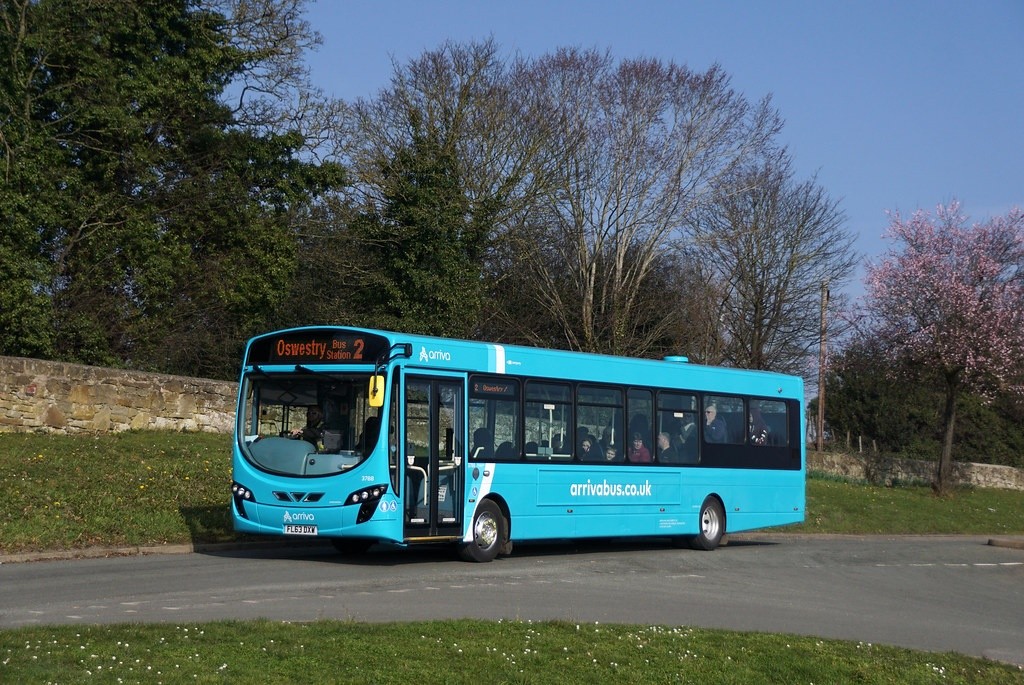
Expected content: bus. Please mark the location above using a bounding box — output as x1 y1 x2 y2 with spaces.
231 323 809 564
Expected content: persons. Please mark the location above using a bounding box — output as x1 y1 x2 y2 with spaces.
654 433 679 465
744 406 769 448
602 445 621 463
676 412 697 453
700 405 730 445
471 416 618 459
627 432 653 465
578 438 601 462
290 405 329 454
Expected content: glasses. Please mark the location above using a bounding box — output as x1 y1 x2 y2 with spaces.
306 411 323 416
